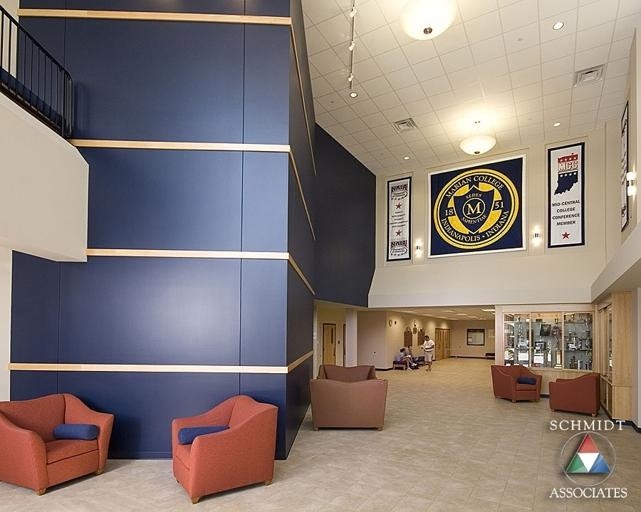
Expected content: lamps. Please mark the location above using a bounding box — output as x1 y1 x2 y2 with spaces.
459 135 497 157
402 1 457 40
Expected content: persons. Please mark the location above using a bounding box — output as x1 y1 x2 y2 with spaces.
420 336 435 371
397 345 418 371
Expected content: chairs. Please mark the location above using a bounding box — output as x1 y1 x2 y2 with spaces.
171 394 279 504
549 371 600 417
0 393 116 496
309 364 388 432
491 364 543 404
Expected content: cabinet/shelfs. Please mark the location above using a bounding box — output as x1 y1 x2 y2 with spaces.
592 291 634 431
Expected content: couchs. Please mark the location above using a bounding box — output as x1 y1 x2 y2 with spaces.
393 355 426 370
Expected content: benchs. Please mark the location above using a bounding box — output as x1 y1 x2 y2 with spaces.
450 355 495 358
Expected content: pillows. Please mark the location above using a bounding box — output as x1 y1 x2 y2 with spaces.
178 424 230 445
52 424 98 440
516 377 536 385
